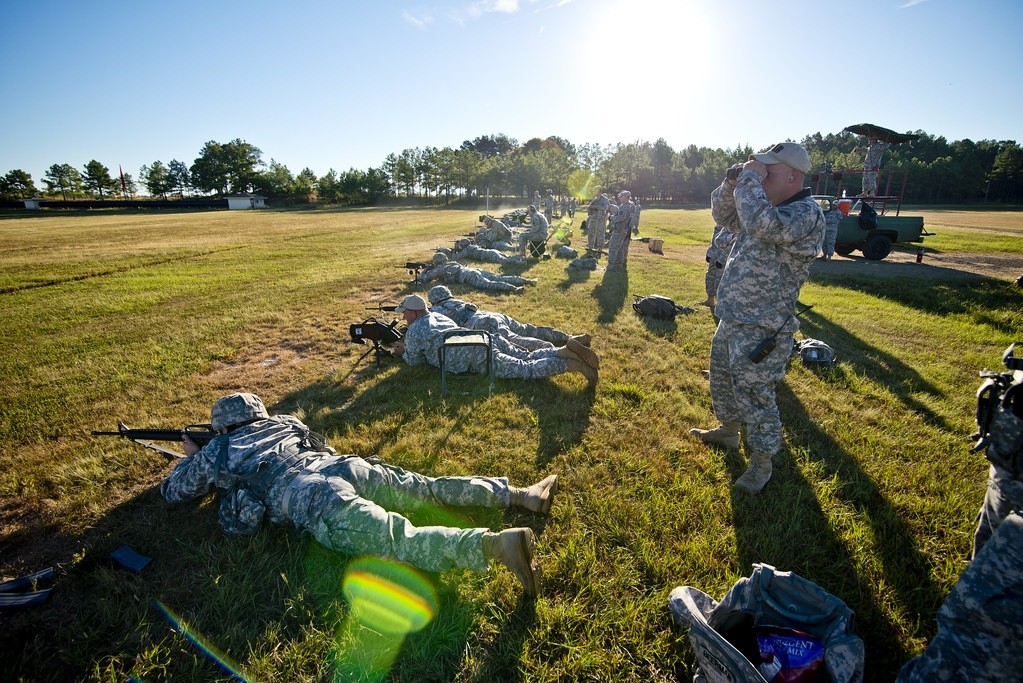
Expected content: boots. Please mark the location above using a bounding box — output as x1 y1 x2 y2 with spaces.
508 474 558 514
556 338 599 368
820 253 827 261
482 526 543 595
689 419 742 449
857 191 869 197
484 234 541 292
734 454 773 492
698 295 714 306
826 255 831 261
868 190 875 198
567 357 599 384
567 333 591 345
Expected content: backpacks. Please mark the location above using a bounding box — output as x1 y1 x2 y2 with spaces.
666 562 864 683
632 294 675 319
558 245 578 260
571 256 598 271
799 338 834 366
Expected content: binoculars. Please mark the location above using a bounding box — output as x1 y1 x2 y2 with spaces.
727 168 742 180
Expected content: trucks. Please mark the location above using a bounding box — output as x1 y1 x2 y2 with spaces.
808 195 936 259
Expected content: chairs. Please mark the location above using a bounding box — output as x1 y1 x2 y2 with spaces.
530 221 562 258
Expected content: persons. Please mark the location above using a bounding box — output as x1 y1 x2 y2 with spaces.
587 186 619 250
519 205 548 256
606 191 641 271
561 194 576 217
427 286 591 352
160 393 559 597
452 239 522 265
891 369 1023 683
855 134 884 198
421 253 537 293
553 199 560 213
476 216 519 251
545 189 554 224
533 190 541 211
394 295 599 385
820 201 842 262
502 209 532 227
688 143 826 494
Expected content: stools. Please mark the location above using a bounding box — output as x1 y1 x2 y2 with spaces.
435 329 494 396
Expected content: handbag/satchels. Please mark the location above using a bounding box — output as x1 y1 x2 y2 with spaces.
216 483 265 535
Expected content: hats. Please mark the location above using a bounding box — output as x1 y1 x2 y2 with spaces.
833 200 838 205
395 295 427 312
748 142 812 176
535 182 640 204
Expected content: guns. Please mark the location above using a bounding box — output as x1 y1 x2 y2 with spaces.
477 226 483 227
461 232 477 237
494 217 505 219
92 422 220 446
449 241 459 242
395 261 427 285
364 303 398 312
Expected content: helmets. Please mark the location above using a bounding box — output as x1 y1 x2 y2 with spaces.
211 206 523 430
